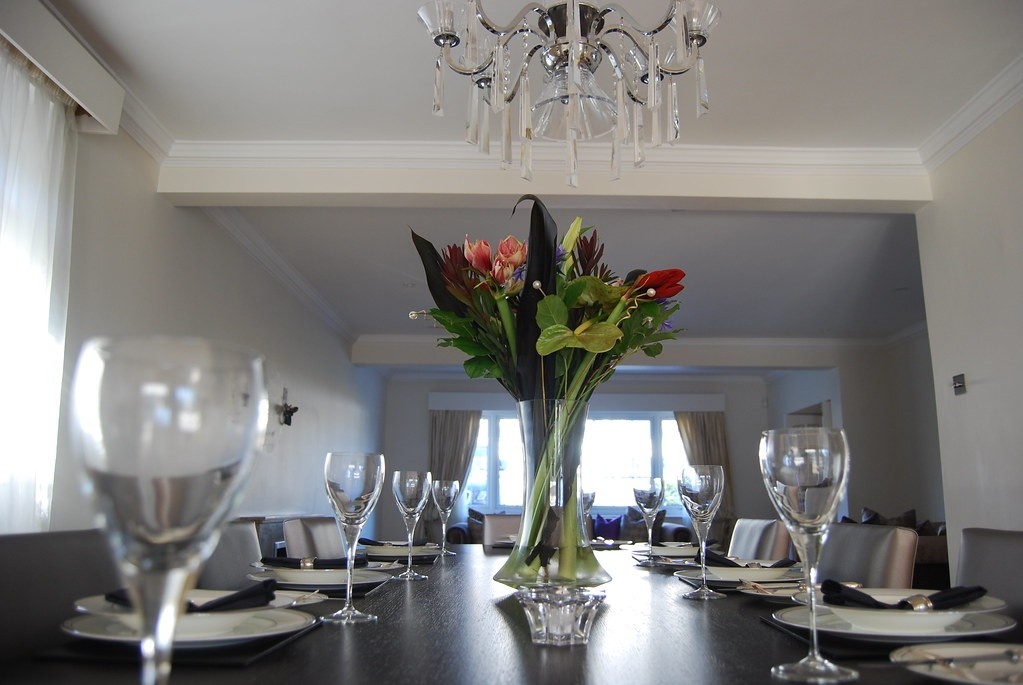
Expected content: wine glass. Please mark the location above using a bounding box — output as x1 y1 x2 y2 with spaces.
632 477 666 568
392 470 432 580
578 477 595 548
318 453 385 624
66 336 269 684
678 465 728 600
758 428 860 683
432 480 460 555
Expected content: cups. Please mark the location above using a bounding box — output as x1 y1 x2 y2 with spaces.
514 588 605 646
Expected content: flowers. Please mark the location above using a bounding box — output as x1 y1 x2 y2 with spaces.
409 194 686 398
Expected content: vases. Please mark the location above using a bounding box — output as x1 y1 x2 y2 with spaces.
493 400 614 588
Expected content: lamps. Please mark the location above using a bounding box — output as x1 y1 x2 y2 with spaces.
417 0 722 188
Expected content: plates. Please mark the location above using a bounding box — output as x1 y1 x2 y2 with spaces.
60 535 1023 685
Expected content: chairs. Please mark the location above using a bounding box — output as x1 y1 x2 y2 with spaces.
1 517 345 653
727 519 1023 642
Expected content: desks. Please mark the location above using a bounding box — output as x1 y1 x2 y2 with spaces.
1 543 1022 685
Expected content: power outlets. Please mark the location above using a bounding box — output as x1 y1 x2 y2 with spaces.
953 374 967 396
